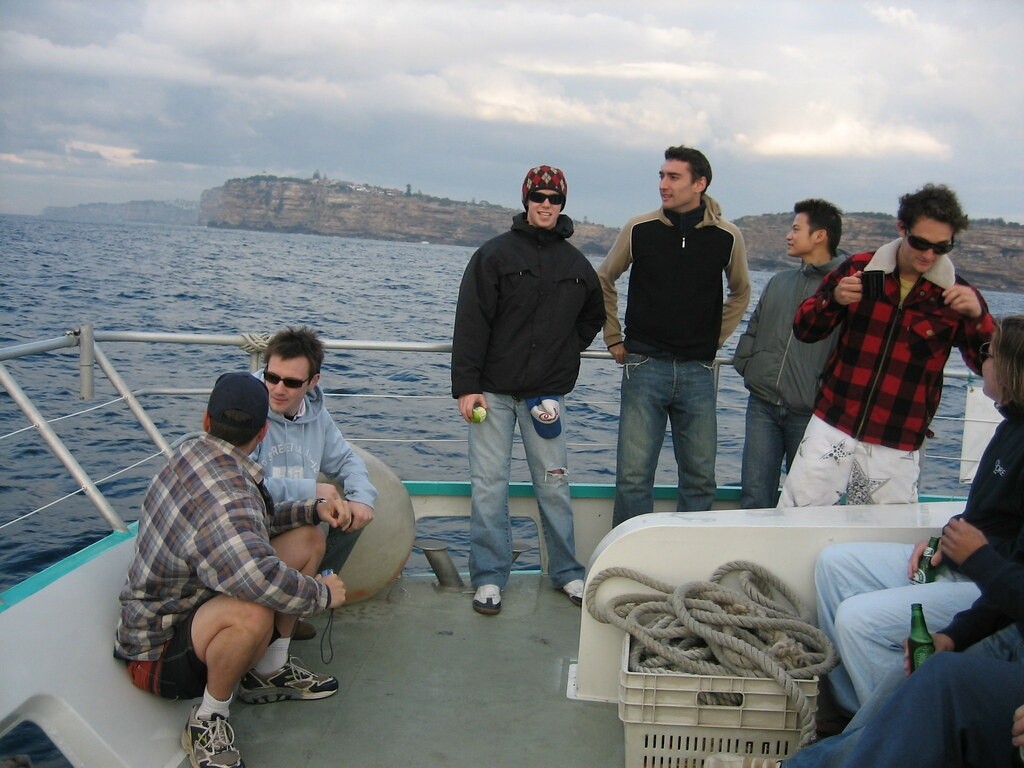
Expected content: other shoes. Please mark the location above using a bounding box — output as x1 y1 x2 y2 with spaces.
292 621 316 639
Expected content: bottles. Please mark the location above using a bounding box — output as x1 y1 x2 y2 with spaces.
909 603 935 676
910 537 939 586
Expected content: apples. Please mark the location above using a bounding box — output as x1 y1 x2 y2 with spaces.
470 406 486 423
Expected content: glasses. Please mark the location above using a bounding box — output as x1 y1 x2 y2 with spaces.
905 226 955 256
528 192 565 205
263 364 310 388
979 342 994 359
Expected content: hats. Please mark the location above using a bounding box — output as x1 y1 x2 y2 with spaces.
527 395 562 439
208 372 270 425
522 166 567 212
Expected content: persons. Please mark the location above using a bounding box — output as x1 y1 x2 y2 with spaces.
733 200 854 509
451 166 606 615
597 146 751 526
250 327 380 640
704 313 1024 768
779 187 996 507
113 372 353 768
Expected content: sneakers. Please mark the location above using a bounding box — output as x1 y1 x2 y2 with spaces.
237 654 339 703
473 584 501 614
181 702 245 768
554 579 585 606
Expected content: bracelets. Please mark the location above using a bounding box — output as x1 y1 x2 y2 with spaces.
318 499 326 503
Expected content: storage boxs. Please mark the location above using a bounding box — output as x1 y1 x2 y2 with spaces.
617 634 819 768
